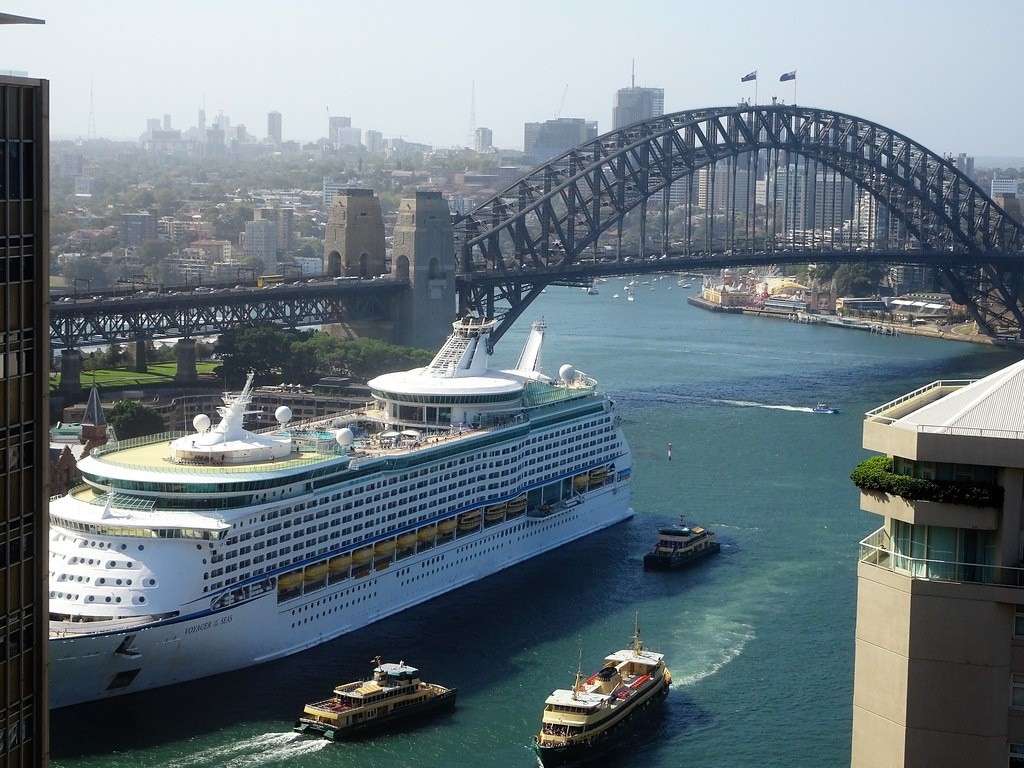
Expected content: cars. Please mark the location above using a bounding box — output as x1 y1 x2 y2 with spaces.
521 242 877 271
52 273 393 304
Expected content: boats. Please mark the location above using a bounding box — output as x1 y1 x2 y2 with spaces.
813 401 840 415
293 654 457 741
585 272 702 301
642 515 721 572
533 612 674 768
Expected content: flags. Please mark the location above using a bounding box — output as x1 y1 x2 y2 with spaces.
780 70 796 81
741 69 757 82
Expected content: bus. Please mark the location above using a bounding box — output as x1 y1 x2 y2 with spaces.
258 274 285 287
333 276 360 284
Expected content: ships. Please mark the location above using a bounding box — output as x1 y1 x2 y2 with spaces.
48 317 635 708
